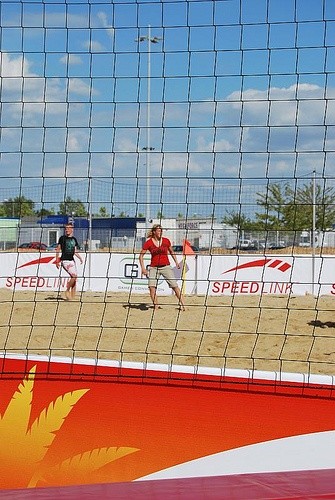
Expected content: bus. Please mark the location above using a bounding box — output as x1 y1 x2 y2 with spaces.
298 236 319 248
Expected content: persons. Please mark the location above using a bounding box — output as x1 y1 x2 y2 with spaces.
56 223 83 300
145 229 155 243
139 223 188 311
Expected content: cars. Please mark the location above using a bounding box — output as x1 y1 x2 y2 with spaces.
18 242 47 251
46 243 58 252
259 240 269 248
269 243 286 250
171 245 199 251
240 240 252 248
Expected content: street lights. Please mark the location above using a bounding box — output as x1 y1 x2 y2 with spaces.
134 24 164 235
